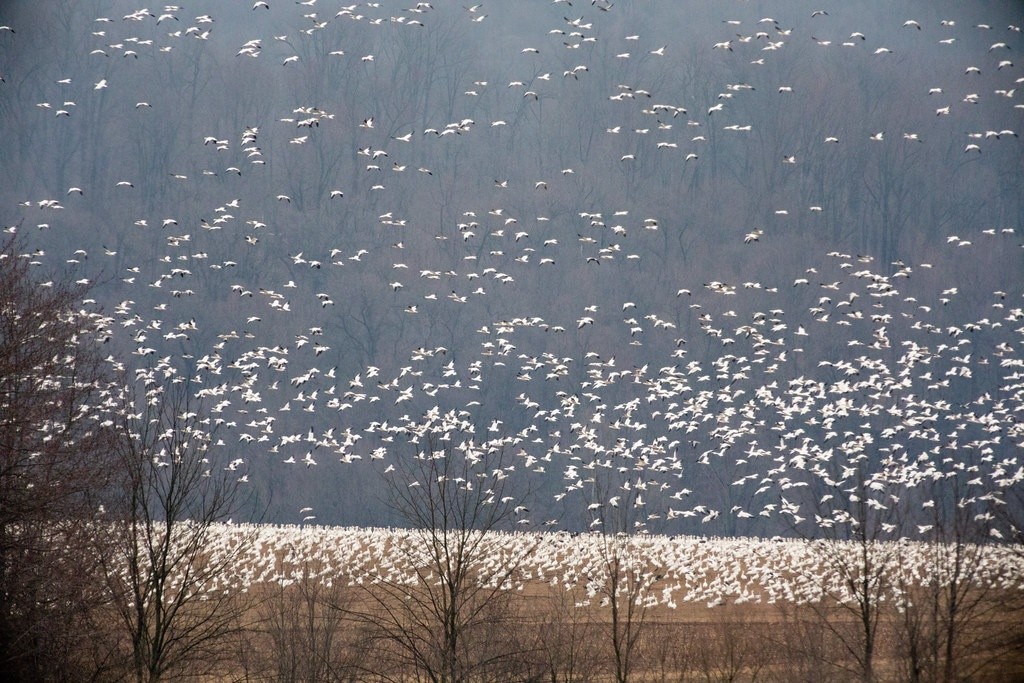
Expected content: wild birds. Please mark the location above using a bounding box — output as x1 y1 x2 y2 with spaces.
1 0 1024 617
